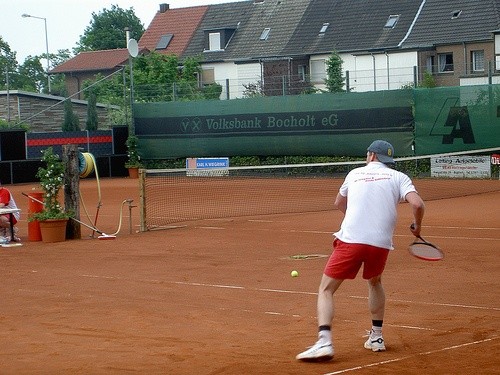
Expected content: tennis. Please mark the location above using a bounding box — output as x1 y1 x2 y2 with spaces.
291 270 300 277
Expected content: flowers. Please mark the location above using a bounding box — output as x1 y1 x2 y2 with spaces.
125 135 142 167
27 148 76 222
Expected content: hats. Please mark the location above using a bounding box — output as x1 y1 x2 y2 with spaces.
368 139 396 164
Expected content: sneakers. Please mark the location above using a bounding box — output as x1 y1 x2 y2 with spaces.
295 341 335 361
362 333 387 351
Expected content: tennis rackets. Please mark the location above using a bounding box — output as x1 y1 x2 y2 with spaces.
406 222 446 261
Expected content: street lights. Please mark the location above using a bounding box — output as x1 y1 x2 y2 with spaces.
21 13 51 95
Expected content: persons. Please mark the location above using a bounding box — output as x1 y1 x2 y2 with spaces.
0 186 20 243
296 139 425 362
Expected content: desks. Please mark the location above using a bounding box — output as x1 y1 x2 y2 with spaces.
0 209 21 245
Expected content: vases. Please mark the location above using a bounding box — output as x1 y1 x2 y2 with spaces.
38 219 67 242
127 168 138 178
